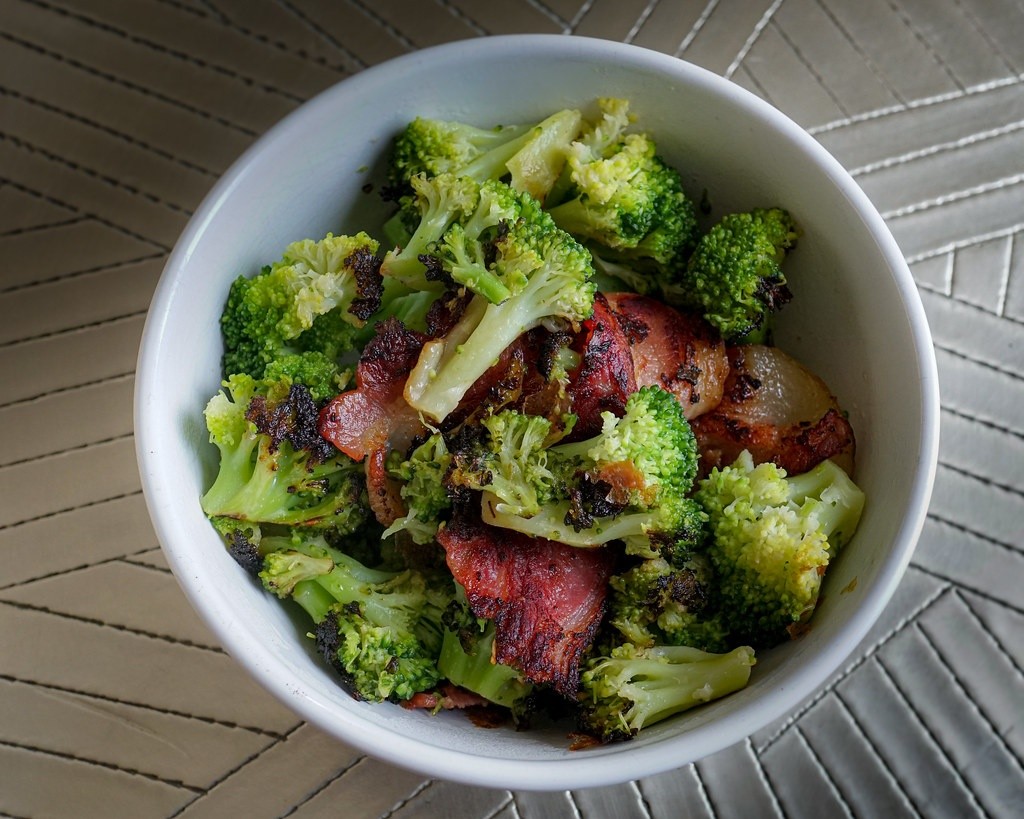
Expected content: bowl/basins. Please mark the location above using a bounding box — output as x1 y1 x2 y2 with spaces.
134 33 940 791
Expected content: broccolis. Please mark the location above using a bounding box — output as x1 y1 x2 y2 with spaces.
199 97 868 748
